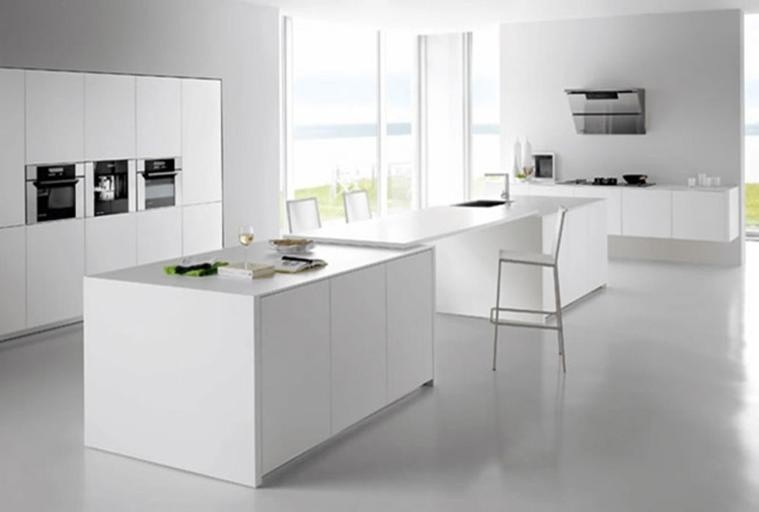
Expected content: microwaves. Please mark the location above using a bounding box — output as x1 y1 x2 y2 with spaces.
533 154 561 180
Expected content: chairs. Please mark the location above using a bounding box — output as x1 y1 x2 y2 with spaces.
490 205 570 372
286 190 371 233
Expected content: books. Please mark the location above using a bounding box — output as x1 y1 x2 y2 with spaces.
217 261 275 280
269 256 327 273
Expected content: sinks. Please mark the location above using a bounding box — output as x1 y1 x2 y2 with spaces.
452 198 511 209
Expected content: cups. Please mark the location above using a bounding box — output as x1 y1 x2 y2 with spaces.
687 174 721 186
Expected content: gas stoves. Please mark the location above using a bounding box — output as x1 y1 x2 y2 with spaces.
558 177 656 187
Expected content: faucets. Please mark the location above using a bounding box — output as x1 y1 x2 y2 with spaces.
483 169 510 205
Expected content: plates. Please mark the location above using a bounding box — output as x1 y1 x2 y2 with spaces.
271 239 314 252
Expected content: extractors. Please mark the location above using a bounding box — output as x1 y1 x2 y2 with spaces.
567 90 646 135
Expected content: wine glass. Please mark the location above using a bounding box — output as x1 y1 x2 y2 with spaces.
238 226 257 267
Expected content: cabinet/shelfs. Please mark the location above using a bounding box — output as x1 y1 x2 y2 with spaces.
509 186 738 244
422 199 607 326
82 251 434 489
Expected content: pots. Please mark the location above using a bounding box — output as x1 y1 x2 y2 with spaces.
624 174 647 183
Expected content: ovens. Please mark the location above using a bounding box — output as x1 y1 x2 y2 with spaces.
23 159 182 226
